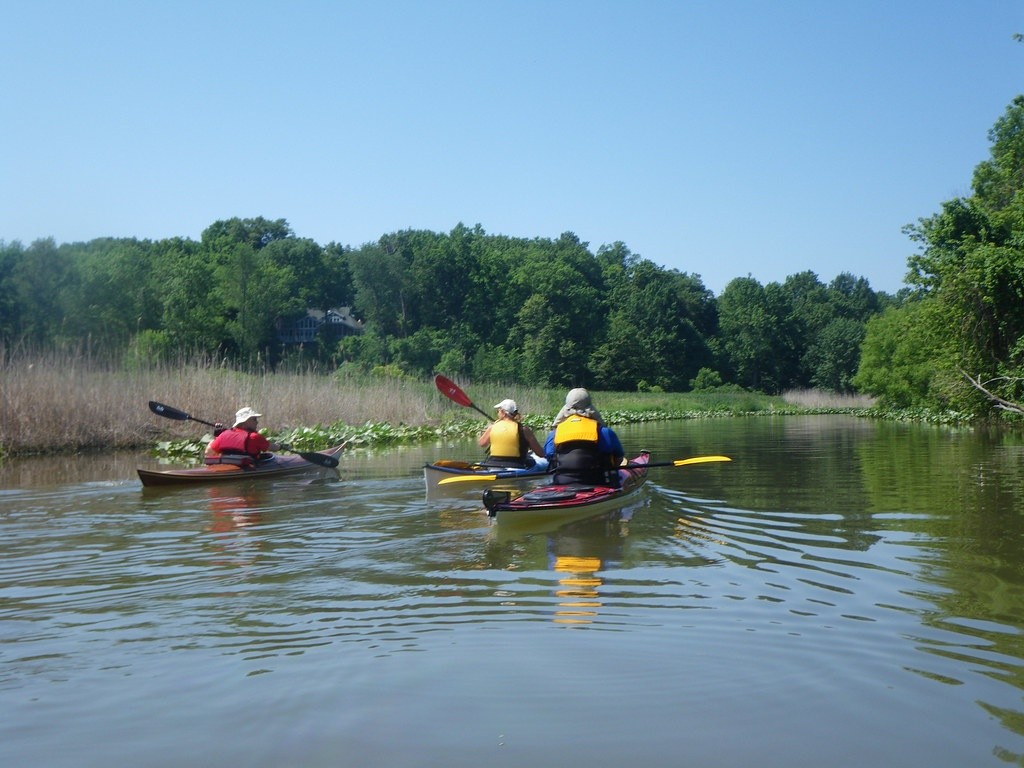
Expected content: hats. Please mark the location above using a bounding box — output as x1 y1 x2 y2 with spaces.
494 399 518 418
232 407 264 428
566 387 590 408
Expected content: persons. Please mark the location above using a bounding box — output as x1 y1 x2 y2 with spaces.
205 406 269 460
544 388 624 488
479 398 545 470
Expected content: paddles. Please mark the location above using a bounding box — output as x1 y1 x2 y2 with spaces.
433 460 505 469
437 455 732 486
435 374 496 422
148 401 339 468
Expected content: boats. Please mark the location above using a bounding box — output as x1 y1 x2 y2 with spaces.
482 449 652 526
135 440 348 496
424 450 549 505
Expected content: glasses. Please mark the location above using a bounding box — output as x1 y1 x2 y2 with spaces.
249 418 256 421
